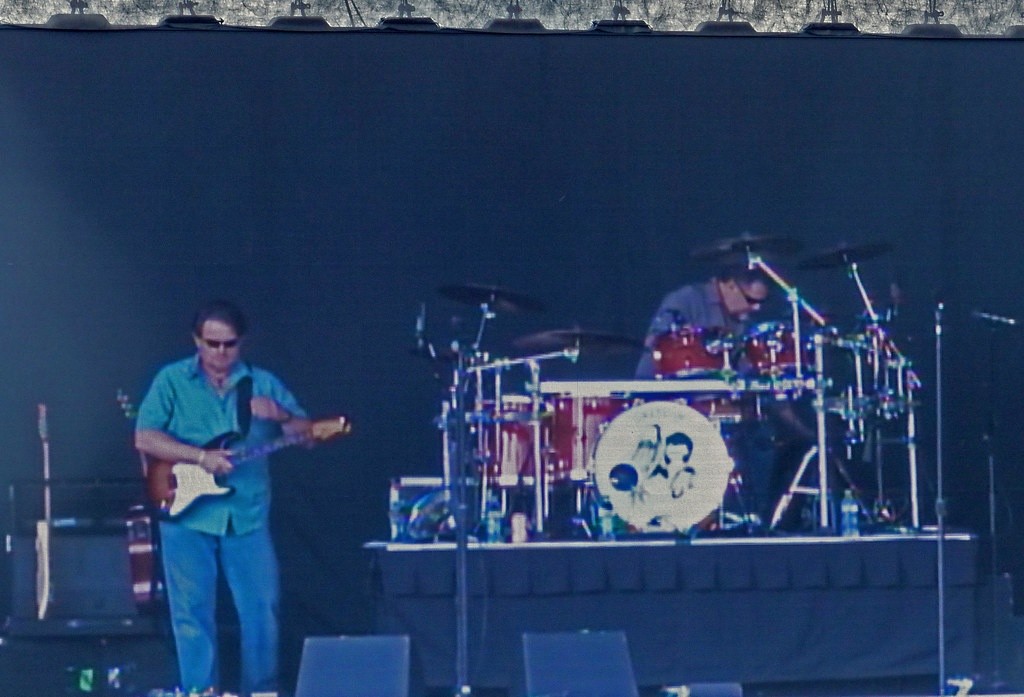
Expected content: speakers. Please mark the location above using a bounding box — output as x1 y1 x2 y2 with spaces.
509 630 640 697
294 635 426 697
691 683 743 697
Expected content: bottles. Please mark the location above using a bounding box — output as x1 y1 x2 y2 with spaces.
841 489 860 538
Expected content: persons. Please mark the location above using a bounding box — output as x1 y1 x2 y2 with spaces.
134 302 315 693
633 262 768 383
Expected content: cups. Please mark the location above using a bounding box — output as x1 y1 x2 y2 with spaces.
511 512 528 543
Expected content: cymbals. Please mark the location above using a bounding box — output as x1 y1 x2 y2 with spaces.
692 235 804 263
512 331 644 360
804 241 893 267
436 280 528 314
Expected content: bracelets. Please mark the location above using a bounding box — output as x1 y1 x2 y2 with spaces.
199 449 205 464
283 411 292 423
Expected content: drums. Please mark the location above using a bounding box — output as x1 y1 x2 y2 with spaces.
473 395 549 487
740 322 817 404
595 403 736 529
651 327 730 379
542 392 634 482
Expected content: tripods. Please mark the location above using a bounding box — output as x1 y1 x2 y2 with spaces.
743 241 879 536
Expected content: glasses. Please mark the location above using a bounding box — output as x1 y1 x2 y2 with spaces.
735 280 766 307
199 335 240 350
